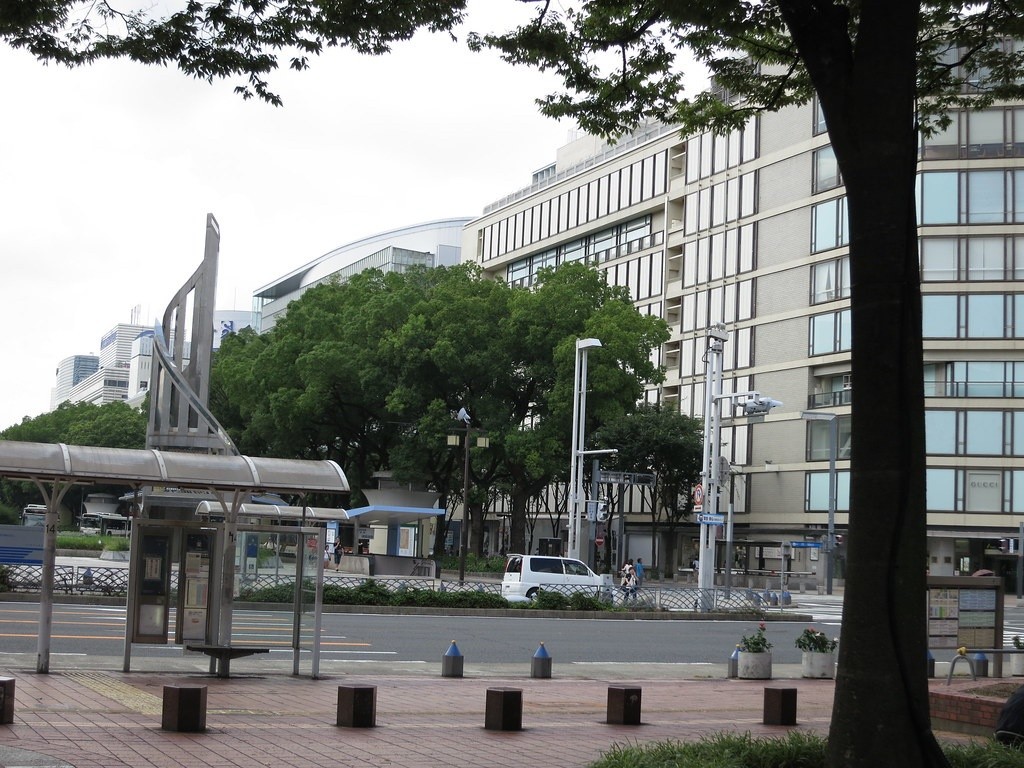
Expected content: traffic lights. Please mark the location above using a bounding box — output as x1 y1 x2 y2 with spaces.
596 501 615 526
782 544 795 555
835 534 845 548
1000 537 1010 555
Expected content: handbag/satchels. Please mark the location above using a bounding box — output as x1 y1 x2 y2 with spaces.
337 553 341 558
329 556 331 561
626 575 636 589
621 574 626 586
693 563 696 569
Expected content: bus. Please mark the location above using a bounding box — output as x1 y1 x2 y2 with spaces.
21 504 47 526
79 512 135 536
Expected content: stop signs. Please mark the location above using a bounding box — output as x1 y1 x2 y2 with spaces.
595 536 604 547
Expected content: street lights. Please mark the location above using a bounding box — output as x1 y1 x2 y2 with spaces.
456 405 472 586
567 338 606 556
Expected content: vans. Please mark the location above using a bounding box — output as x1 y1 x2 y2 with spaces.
501 553 616 603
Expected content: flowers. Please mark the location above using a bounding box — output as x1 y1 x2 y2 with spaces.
740 623 774 653
794 627 839 653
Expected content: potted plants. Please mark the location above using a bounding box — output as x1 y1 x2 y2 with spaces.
1010 635 1024 676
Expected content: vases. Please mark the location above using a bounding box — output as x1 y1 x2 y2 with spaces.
801 651 835 678
738 651 772 679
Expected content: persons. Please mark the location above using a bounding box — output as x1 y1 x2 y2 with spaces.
737 551 744 569
620 558 645 603
358 538 369 555
692 558 700 570
333 536 344 572
264 536 277 551
500 545 508 556
324 545 331 569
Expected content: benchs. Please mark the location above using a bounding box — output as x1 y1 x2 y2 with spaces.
187 644 270 677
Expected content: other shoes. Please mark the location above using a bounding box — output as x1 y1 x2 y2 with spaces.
336 569 338 571
